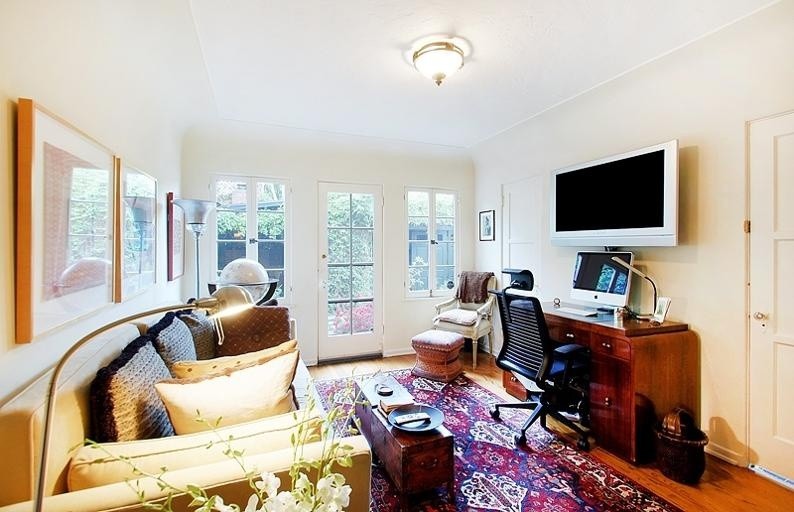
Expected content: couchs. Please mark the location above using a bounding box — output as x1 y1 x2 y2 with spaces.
0 285 373 511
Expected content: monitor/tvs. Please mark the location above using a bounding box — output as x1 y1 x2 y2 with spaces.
571 251 634 314
549 138 679 247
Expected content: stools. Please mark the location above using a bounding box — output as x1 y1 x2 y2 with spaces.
410 330 465 383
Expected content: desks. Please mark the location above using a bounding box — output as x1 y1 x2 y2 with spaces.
503 301 701 466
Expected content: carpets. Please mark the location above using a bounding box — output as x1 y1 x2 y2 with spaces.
314 369 684 512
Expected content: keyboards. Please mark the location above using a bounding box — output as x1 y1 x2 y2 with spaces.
556 306 598 317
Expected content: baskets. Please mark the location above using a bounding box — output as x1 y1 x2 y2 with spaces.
655 408 709 485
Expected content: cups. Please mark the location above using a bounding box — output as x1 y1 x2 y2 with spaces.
613 307 624 320
614 320 624 328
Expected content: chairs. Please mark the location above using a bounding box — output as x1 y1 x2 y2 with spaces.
488 268 596 450
434 271 496 370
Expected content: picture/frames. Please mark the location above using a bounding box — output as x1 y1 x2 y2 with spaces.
652 297 671 322
479 210 495 241
168 192 186 281
116 158 158 302
16 98 116 343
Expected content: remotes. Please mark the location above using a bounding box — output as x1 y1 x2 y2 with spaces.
393 412 431 426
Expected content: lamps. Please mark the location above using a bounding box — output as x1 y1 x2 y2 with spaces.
171 198 221 298
403 33 473 85
611 256 657 322
35 285 254 511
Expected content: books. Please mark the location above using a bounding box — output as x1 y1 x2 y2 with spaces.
378 395 414 419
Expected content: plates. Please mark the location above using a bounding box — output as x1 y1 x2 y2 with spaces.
387 404 445 433
